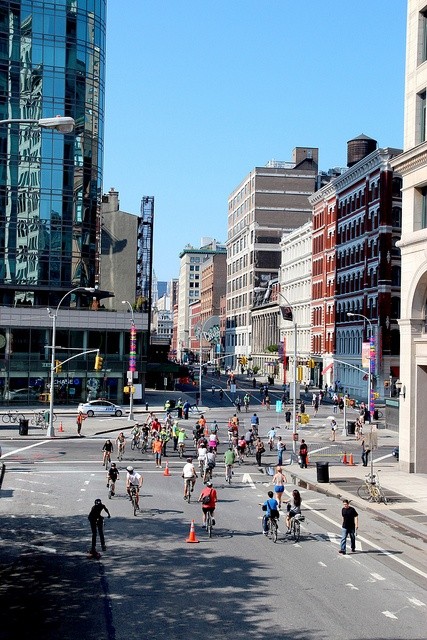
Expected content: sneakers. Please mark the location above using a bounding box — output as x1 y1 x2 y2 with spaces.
102 546 106 551
202 524 205 528
339 549 346 554
129 499 133 501
137 506 139 510
183 496 187 500
212 519 215 525
90 548 96 554
191 489 193 492
351 548 355 551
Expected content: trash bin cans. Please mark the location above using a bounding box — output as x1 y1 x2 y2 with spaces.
348 421 356 434
196 393 200 400
19 419 29 436
315 462 330 483
170 400 176 408
374 410 378 420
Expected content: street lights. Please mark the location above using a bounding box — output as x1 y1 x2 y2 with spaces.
0 112 76 227
346 313 373 422
121 300 136 420
197 315 226 407
252 286 299 453
46 286 97 437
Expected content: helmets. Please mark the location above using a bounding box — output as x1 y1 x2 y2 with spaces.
111 463 116 467
126 466 134 471
186 458 192 461
200 443 204 445
207 482 213 487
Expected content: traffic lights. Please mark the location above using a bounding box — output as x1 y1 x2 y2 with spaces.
309 360 316 369
239 357 249 367
94 356 103 369
38 393 47 403
53 360 64 374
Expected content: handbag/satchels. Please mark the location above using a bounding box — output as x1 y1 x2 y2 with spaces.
258 445 265 452
206 453 215 469
202 496 211 504
270 509 279 519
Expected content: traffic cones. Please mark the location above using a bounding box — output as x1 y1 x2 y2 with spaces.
162 460 172 478
185 519 199 543
305 455 310 466
347 453 357 466
342 450 349 465
58 419 64 432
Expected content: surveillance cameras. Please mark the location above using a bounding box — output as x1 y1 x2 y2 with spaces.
46 308 52 315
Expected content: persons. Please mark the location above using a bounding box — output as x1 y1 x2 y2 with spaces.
267 426 276 451
339 500 358 554
276 436 286 467
197 480 218 528
107 462 119 495
268 373 371 417
299 439 309 469
261 491 279 536
181 458 199 500
116 431 127 459
223 448 235 481
285 407 292 428
129 352 268 467
88 499 111 553
331 419 337 442
100 438 113 466
353 417 366 441
282 489 302 534
272 466 287 506
125 465 144 509
361 440 371 467
76 411 83 436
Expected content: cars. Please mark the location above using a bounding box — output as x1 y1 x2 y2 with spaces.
77 400 127 418
5 386 39 404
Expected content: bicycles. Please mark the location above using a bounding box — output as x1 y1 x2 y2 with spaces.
140 436 147 454
268 435 275 450
181 474 193 504
116 443 125 461
204 464 212 483
198 499 216 538
131 433 140 451
258 502 280 543
357 472 381 504
223 457 234 485
30 409 44 426
106 478 119 499
178 441 185 459
1 410 25 423
369 469 389 506
246 438 257 457
237 449 244 466
282 501 305 543
196 454 207 478
150 429 157 450
127 486 141 516
38 410 57 423
101 450 111 470
